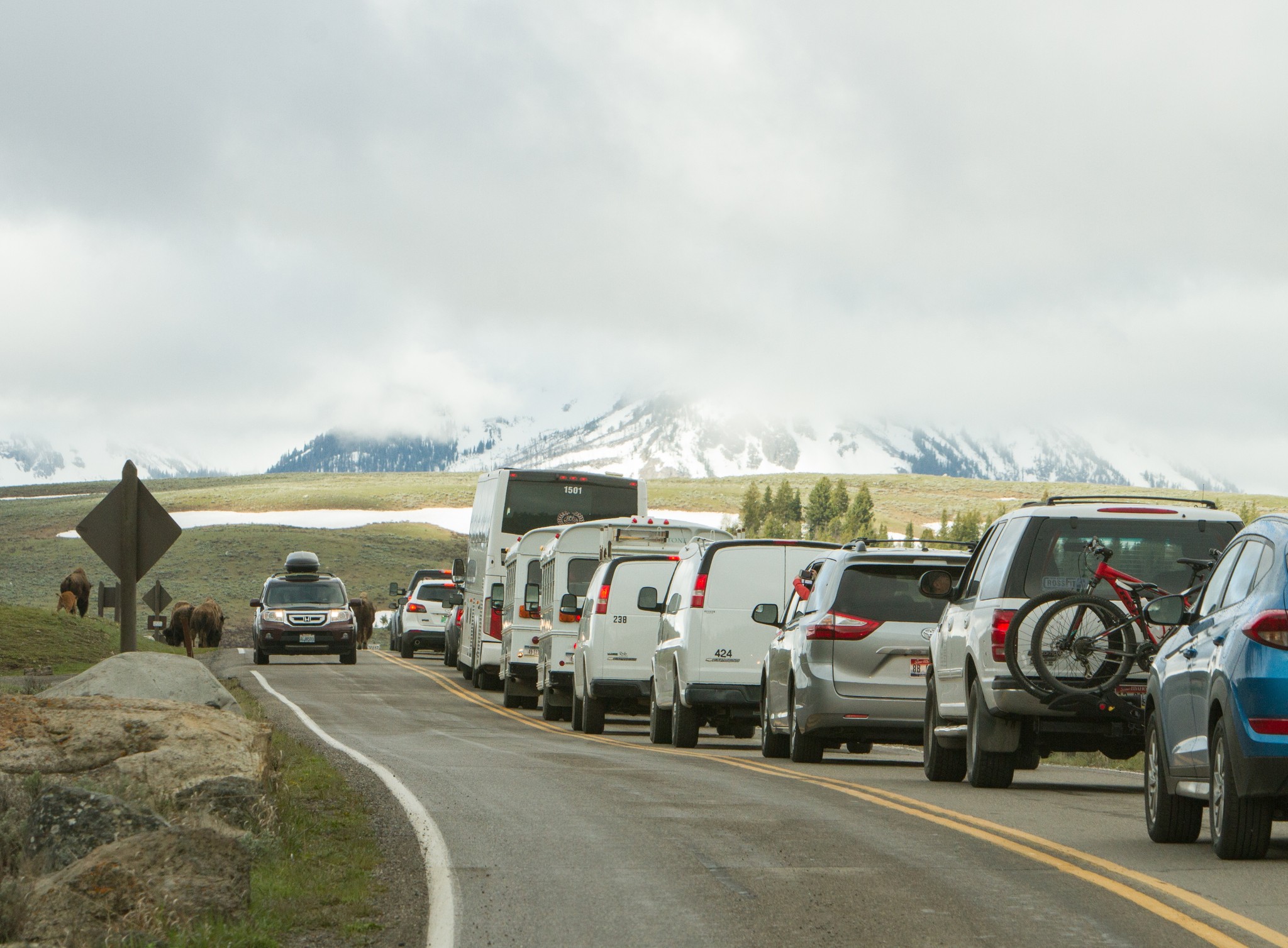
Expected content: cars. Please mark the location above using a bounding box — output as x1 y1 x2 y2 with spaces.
442 583 472 668
1143 513 1288 860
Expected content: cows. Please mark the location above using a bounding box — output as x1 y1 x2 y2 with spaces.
352 592 377 650
55 567 95 619
162 597 230 648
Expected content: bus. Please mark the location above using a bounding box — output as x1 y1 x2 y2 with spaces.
451 467 647 691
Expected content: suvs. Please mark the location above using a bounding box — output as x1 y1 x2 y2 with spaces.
248 551 360 664
389 569 460 653
917 495 1252 791
752 536 982 762
397 579 466 658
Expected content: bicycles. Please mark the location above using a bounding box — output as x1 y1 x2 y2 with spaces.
1030 535 1224 700
1004 536 1219 702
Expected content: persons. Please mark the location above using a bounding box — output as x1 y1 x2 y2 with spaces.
928 571 950 594
793 569 818 600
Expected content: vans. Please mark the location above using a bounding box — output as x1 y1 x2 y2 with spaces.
489 522 579 710
637 536 858 748
523 515 735 722
558 555 682 738
